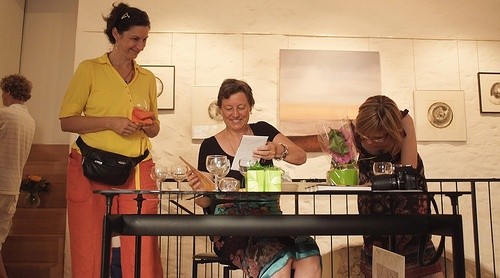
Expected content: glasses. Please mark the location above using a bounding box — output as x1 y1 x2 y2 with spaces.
358 132 389 144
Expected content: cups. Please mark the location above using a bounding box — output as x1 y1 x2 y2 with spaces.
373 162 394 176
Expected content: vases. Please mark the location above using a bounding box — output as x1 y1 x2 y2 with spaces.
24 191 41 208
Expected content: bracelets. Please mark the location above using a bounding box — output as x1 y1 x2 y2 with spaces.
274 143 290 161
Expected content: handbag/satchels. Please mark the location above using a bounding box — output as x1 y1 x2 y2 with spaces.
76 136 150 187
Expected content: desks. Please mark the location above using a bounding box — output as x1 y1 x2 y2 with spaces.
93 187 473 278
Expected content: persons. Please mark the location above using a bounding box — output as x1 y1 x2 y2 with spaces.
57 3 164 277
186 78 322 278
0 73 36 277
325 95 444 278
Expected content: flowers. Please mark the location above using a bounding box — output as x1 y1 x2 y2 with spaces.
21 174 50 194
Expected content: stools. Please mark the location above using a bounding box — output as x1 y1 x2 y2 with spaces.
193 252 243 278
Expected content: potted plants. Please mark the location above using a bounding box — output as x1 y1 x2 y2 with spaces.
325 125 360 187
264 163 282 192
245 161 266 192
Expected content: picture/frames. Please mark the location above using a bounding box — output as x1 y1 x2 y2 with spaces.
412 90 468 142
478 71 500 114
140 64 176 110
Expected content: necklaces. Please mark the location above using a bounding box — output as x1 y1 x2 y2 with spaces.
224 125 251 155
108 50 133 83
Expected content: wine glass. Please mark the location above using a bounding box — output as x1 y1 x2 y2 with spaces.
170 165 189 191
151 165 168 191
206 155 228 191
134 99 148 136
215 159 231 184
238 158 259 187
